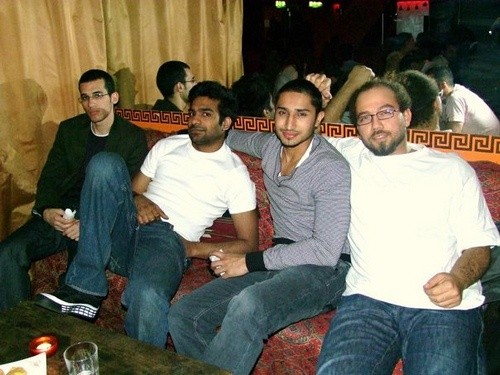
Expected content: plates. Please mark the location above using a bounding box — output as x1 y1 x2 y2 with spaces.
0 352 47 375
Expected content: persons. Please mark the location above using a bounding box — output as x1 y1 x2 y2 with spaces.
152 60 197 112
35 81 259 348
231 32 500 136
0 69 148 307
166 78 351 375
305 73 500 375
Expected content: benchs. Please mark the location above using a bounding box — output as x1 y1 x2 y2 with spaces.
32 130 500 375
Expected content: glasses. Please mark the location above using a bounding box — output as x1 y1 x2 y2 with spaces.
354 108 400 126
181 79 195 83
79 92 112 102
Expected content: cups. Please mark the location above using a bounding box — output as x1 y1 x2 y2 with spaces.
63 342 99 375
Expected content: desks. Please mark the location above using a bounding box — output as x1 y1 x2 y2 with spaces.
0 301 234 375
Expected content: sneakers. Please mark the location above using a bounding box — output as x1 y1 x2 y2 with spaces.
35 284 101 319
